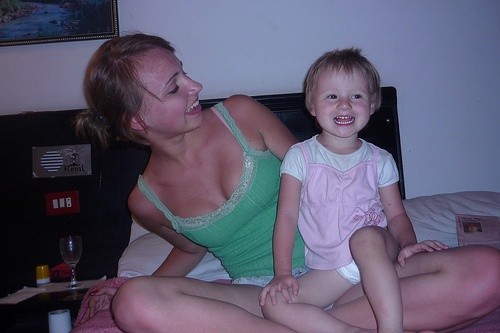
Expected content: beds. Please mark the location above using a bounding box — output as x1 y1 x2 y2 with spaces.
0 87 500 332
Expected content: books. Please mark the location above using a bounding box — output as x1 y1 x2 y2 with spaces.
454 213 500 247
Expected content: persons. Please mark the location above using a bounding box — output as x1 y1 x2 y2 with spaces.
75 33 500 333
258 43 449 333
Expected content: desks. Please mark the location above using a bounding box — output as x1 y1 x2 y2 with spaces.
0 256 113 333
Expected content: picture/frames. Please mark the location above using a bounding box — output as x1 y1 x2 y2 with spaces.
0 0 119 45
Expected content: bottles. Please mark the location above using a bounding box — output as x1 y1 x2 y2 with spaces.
36 265 51 284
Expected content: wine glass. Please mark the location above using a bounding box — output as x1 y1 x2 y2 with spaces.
60 236 86 289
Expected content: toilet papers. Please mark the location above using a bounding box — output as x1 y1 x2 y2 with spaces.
49 310 73 333
